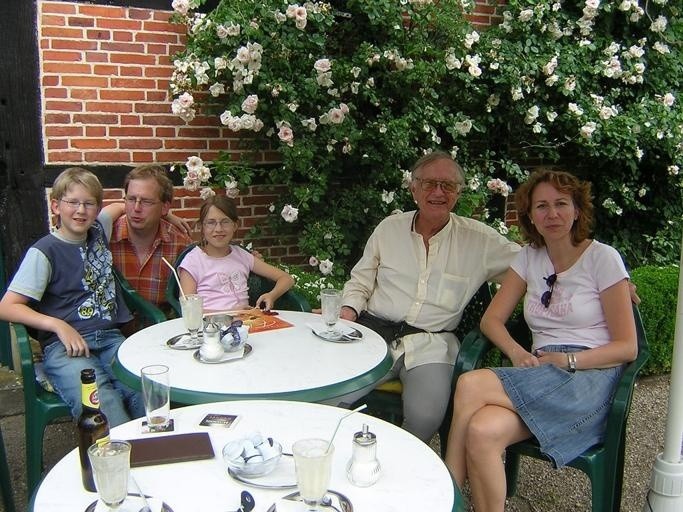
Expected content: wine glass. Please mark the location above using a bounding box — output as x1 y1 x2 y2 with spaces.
321 289 344 339
87 440 133 507
179 294 205 347
291 437 335 512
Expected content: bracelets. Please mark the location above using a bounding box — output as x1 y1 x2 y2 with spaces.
566 352 577 373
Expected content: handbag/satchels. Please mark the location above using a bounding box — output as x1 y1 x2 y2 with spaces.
353 310 424 344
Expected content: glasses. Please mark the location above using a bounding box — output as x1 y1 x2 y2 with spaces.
415 178 461 193
541 275 556 308
203 219 232 228
123 197 161 207
59 199 96 208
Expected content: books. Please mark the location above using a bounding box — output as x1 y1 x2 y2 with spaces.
113 431 215 468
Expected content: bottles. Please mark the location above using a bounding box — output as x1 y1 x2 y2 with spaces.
346 423 382 489
198 321 224 361
76 368 111 493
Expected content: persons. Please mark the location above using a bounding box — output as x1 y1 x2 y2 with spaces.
105 163 265 338
178 193 296 313
443 165 639 512
0 165 147 429
313 151 642 445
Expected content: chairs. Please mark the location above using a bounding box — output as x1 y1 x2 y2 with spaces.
351 270 491 460
11 266 166 509
461 302 650 512
166 237 314 316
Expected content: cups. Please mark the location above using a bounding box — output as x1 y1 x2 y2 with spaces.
141 365 171 429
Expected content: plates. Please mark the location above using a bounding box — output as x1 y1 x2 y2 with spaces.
166 330 203 349
193 343 252 364
227 453 299 487
312 325 362 343
84 493 174 512
266 490 354 512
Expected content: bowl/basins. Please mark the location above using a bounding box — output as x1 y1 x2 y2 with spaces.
222 437 283 478
203 314 233 327
